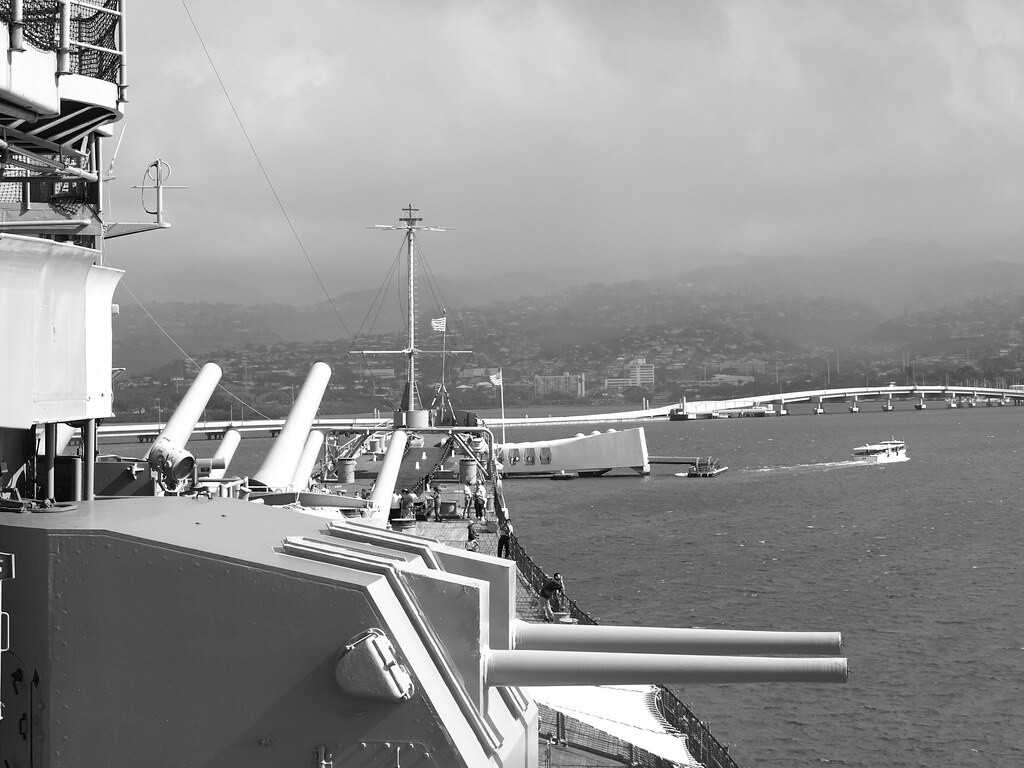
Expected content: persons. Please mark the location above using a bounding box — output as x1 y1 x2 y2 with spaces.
462 481 473 520
388 489 417 524
497 518 513 559
310 475 376 500
510 456 520 465
465 520 480 553
473 480 486 522
540 572 564 621
430 487 443 522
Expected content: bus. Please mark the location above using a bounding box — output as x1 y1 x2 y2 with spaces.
1008 385 1024 391
883 381 896 387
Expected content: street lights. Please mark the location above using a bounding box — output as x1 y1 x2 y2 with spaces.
291 384 300 403
861 373 869 391
155 399 161 430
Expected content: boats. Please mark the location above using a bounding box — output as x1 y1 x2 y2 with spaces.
851 433 907 463
686 457 728 479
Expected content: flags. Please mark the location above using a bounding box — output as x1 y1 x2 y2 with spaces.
431 318 446 332
489 372 501 385
